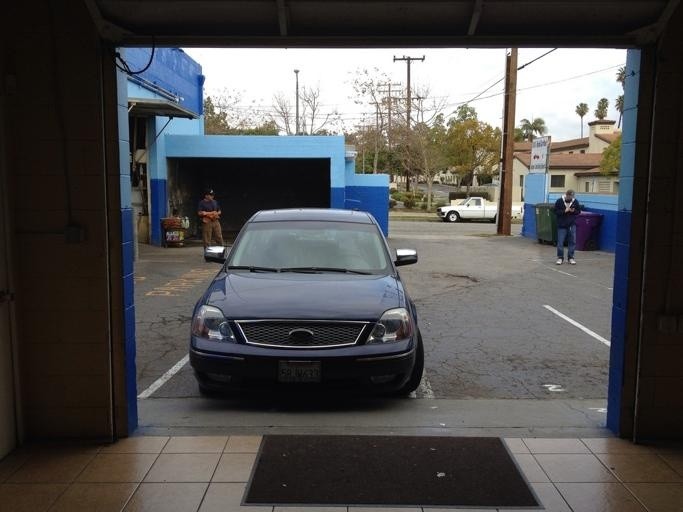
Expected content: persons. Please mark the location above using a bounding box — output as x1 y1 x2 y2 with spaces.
196 189 223 249
553 190 581 265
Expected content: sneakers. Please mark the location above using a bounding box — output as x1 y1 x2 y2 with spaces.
556 259 563 264
568 258 575 264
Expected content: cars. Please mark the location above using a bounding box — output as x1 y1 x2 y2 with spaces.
189 206 427 402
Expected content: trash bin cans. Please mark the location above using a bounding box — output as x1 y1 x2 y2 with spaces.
575 210 603 251
535 203 585 247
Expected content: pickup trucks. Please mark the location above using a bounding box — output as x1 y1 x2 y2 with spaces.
434 195 521 223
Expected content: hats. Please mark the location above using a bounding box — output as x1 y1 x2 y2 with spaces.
203 188 215 196
566 190 574 197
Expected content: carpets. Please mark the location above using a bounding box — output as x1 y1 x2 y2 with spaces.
240 432 546 506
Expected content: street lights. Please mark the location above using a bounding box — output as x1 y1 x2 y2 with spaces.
294 68 301 137
